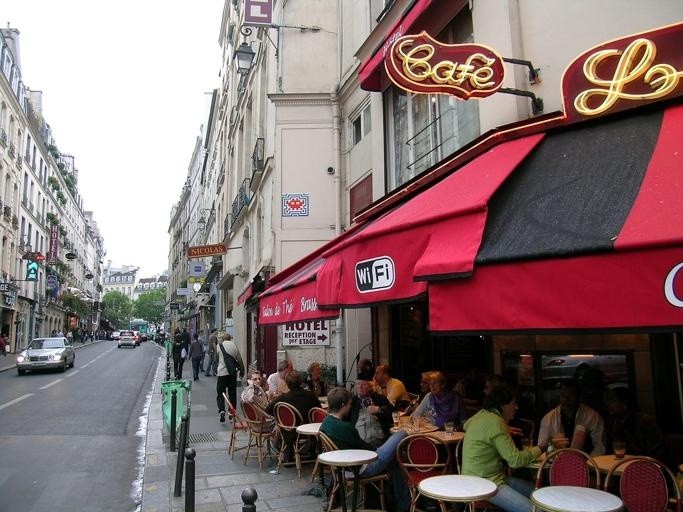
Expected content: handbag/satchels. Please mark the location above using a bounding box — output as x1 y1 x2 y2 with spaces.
355 413 386 444
223 352 238 378
180 348 188 360
202 344 207 353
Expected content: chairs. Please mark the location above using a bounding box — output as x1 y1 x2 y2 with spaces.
392 433 453 510
529 447 601 512
313 430 391 512
237 399 278 473
455 431 494 512
222 391 265 462
273 401 307 479
603 455 681 512
309 408 329 425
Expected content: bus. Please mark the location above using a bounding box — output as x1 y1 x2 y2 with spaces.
130 319 148 341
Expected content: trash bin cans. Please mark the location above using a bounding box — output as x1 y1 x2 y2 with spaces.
160 379 192 433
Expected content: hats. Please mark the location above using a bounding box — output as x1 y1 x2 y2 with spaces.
357 372 374 382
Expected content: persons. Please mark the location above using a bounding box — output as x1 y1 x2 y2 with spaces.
215 333 245 423
172 326 220 381
239 358 663 511
0 328 112 356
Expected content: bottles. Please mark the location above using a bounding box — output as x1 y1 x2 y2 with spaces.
547 437 556 463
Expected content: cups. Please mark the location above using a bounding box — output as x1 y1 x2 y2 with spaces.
412 416 423 432
392 413 402 430
612 440 625 458
445 420 454 434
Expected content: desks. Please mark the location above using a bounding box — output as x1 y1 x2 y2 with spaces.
515 442 571 474
586 451 662 483
312 393 468 459
410 474 500 511
530 483 625 512
294 422 327 477
316 448 379 511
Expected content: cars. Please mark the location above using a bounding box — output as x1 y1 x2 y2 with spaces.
111 330 142 348
16 337 75 375
542 355 627 390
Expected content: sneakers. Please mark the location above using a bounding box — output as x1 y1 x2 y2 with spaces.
220 413 226 422
